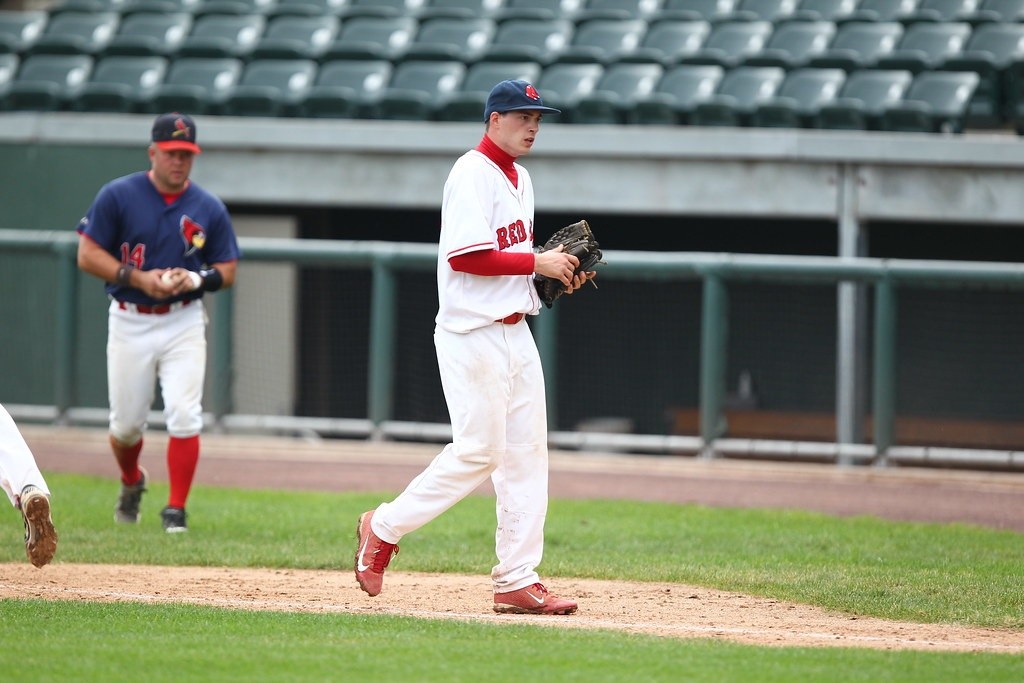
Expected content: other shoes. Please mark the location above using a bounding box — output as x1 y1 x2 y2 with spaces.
18 484 58 568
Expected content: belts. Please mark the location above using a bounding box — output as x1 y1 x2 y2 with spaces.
494 312 523 325
117 300 191 315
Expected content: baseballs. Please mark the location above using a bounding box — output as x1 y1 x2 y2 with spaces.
157 265 184 299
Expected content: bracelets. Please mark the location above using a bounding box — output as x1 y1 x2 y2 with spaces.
117 263 134 287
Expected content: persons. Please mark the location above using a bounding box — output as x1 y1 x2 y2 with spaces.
0 403 58 568
354 79 597 616
76 112 241 536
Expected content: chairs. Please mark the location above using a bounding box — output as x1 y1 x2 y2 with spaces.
0 0 1024 136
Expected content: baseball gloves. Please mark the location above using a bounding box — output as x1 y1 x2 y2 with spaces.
525 220 605 307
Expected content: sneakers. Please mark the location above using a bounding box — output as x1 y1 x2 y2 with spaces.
113 467 147 522
493 582 578 615
160 505 188 533
353 508 399 596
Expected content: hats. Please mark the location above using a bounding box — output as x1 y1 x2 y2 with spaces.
484 78 561 115
152 111 202 155
